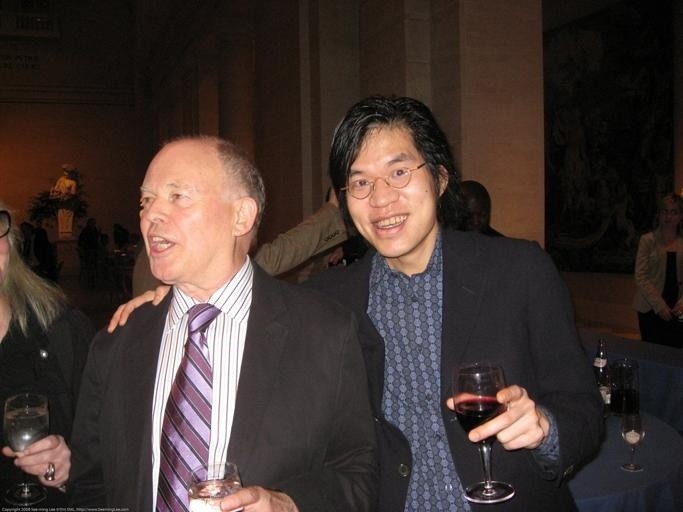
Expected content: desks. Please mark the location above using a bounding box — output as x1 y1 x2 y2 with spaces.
566 409 683 512
581 332 681 431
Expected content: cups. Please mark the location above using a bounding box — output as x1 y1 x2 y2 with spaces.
186 462 243 512
611 358 640 417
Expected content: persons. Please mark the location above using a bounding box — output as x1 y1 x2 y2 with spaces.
436 179 504 239
66 133 392 512
106 93 607 510
131 186 353 312
631 193 682 350
16 216 141 304
0 195 100 512
326 230 367 268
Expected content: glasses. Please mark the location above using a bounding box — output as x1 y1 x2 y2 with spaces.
0 209 12 238
339 158 429 201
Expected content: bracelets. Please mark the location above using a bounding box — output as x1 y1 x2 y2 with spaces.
42 461 54 481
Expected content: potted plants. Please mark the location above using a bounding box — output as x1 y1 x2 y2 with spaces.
24 167 91 232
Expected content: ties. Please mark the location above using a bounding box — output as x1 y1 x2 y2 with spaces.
153 301 224 512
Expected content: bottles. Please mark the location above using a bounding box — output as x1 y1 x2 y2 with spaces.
590 338 612 416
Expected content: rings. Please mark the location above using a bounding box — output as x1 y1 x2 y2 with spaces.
678 310 682 314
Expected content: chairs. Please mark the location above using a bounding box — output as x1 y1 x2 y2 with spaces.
19 219 139 329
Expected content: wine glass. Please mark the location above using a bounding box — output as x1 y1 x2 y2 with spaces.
450 362 519 506
3 392 51 500
618 415 647 474
